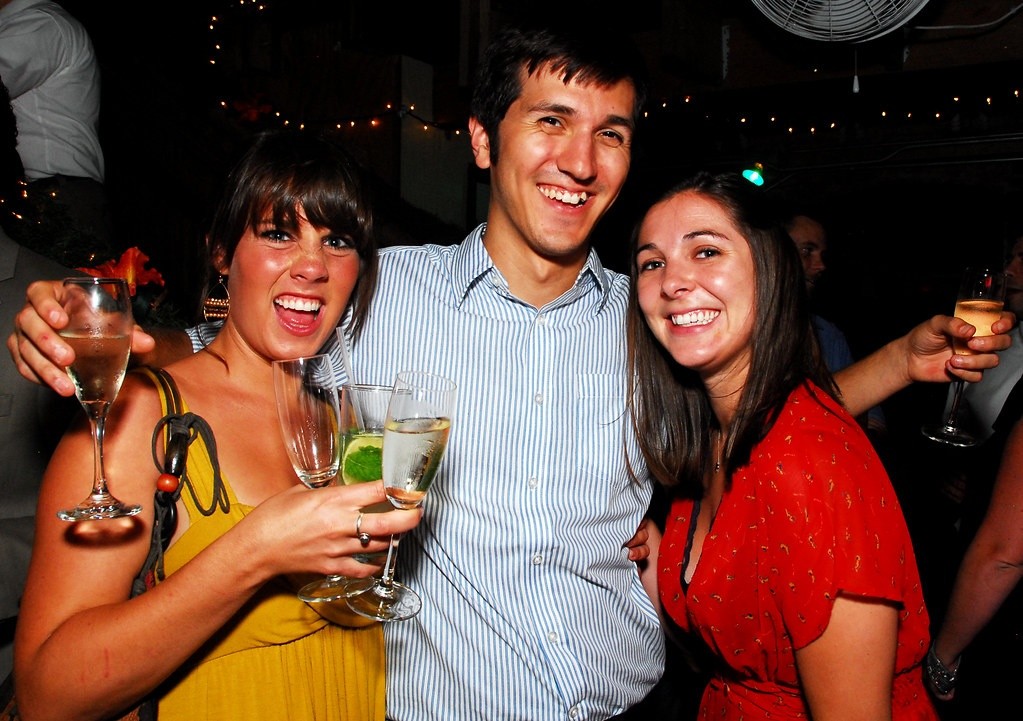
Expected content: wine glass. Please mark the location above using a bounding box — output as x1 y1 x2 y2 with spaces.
57 276 144 520
341 371 457 622
272 353 377 603
921 261 1009 447
336 383 393 565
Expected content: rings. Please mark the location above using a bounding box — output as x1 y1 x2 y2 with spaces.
356 513 370 544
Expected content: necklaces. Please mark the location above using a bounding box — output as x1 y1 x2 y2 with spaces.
714 430 724 475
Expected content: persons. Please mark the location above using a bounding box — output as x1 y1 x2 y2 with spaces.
943 240 1023 506
8 18 1014 721
920 416 1022 701
783 207 883 442
15 124 424 721
0 0 103 267
620 169 936 721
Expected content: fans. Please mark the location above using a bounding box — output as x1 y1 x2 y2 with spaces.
751 0 930 45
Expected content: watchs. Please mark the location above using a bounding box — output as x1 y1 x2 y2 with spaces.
924 643 961 694
